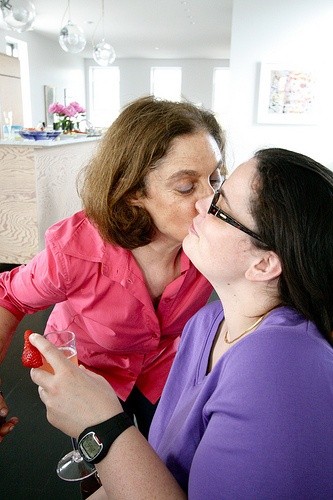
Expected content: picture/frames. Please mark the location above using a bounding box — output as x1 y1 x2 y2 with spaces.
44 85 54 127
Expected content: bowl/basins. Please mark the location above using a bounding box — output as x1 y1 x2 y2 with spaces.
19 131 61 142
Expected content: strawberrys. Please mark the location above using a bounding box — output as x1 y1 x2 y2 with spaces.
21 329 43 368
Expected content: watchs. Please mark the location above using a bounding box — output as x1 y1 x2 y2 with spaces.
77 412 135 464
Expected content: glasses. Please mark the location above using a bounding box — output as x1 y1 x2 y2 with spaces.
208 179 277 251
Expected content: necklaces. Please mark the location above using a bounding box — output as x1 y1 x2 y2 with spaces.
224 314 265 344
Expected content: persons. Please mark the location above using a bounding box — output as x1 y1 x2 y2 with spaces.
30 148 333 500
0 96 228 440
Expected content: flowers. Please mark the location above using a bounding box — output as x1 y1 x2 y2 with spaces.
48 100 87 136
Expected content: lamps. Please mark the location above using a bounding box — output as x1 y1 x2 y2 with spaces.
57 0 87 56
91 0 117 67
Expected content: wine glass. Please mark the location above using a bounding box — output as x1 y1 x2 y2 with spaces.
3 111 13 134
37 331 97 482
